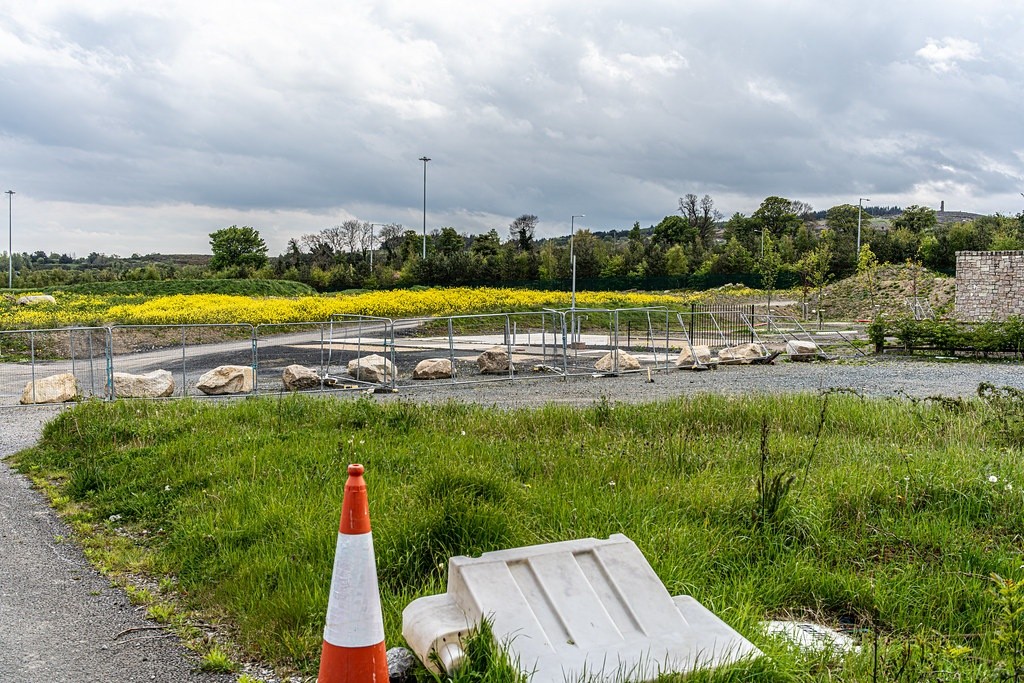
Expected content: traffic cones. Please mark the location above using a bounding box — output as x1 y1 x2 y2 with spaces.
313 466 394 683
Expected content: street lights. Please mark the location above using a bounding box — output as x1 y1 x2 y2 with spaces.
418 156 432 260
754 230 763 258
368 223 386 276
571 215 586 266
854 198 871 260
5 189 16 290
